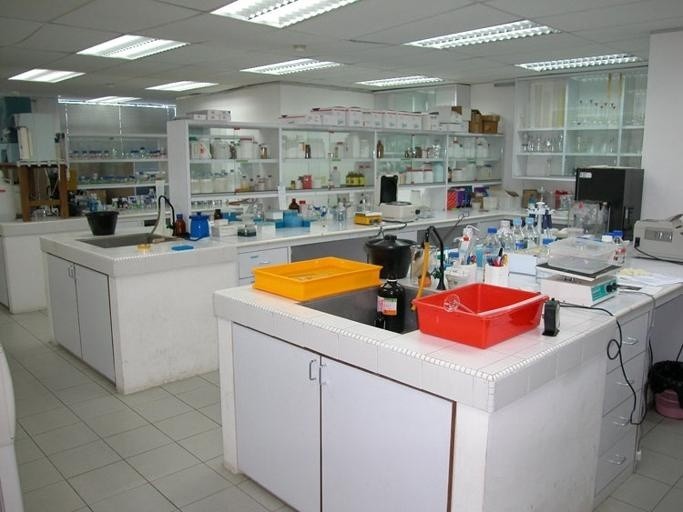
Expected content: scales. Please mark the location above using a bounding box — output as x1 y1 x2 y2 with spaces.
535 262 622 308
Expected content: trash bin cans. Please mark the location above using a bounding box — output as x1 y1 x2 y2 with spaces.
651 360 683 420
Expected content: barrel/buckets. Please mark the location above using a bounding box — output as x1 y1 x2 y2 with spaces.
0 170 17 221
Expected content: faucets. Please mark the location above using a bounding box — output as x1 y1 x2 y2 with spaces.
424 225 445 290
158 194 177 235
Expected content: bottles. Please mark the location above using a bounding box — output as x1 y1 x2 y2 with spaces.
213 208 224 220
344 169 367 187
68 144 167 213
455 215 540 265
377 138 385 158
612 230 626 265
287 197 376 230
174 211 187 236
238 172 278 193
371 273 408 334
409 244 439 287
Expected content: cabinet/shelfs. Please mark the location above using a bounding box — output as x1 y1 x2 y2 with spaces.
238 246 291 287
514 68 644 211
165 118 506 231
232 322 455 512
63 128 168 192
45 253 116 384
594 310 649 495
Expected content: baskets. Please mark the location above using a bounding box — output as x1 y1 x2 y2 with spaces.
469 109 500 134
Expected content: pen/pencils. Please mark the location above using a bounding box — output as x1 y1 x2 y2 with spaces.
487 248 507 267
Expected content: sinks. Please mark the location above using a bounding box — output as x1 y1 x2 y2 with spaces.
294 282 447 336
75 229 176 250
